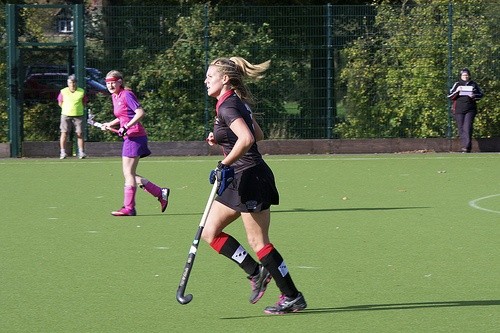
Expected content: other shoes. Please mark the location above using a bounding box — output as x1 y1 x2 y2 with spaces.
461 148 471 153
157 188 170 213
112 206 136 216
79 152 86 159
60 153 68 159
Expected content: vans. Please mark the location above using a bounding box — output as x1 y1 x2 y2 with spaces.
22 73 108 106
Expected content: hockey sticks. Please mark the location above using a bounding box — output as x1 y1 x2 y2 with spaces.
175 177 221 305
87 113 127 136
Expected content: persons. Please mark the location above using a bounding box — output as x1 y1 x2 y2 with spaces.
58 75 88 160
101 70 170 216
201 57 308 315
446 68 483 154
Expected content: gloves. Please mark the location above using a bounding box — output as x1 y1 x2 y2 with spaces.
209 161 234 196
118 124 128 137
101 122 111 130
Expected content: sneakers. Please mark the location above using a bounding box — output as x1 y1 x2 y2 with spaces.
264 291 307 315
247 265 272 304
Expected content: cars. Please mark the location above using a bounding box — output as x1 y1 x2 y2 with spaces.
84 68 104 79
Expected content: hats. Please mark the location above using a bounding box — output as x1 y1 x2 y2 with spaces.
67 73 77 82
